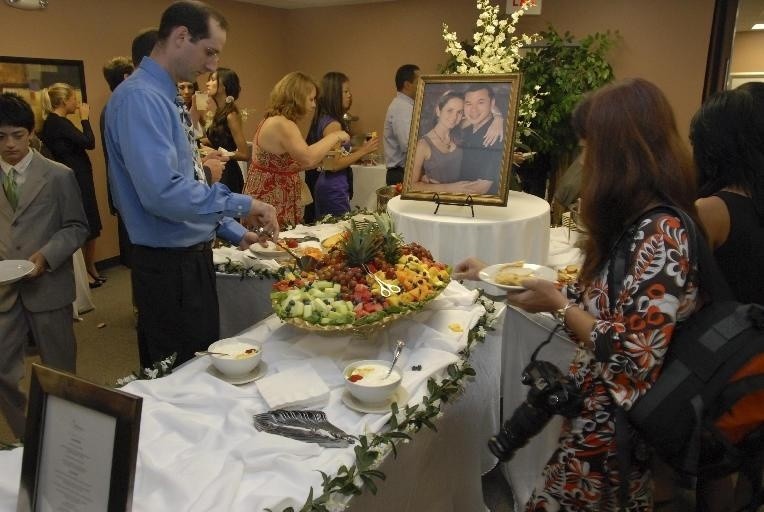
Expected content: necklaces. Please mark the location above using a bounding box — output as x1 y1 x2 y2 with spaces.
431 128 451 149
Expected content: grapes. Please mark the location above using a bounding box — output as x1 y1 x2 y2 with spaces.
331 259 394 304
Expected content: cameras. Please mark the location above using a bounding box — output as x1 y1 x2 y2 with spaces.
489 360 585 463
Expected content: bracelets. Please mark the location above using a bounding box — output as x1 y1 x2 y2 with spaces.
493 112 503 117
554 300 579 327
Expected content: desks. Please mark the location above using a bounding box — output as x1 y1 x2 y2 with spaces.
386 190 551 297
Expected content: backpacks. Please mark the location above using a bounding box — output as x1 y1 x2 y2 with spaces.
610 199 763 480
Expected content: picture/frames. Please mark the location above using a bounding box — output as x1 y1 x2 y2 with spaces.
506 0 542 15
0 56 87 133
401 72 523 207
16 362 143 512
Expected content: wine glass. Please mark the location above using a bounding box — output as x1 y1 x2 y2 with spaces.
363 128 380 160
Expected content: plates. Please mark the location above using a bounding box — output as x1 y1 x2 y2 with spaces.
214 150 237 157
0 260 36 286
203 359 269 385
247 238 301 256
342 386 418 414
477 262 559 292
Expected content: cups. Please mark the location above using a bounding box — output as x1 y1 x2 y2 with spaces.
195 91 209 112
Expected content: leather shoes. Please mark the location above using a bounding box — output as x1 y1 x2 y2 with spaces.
88 275 108 288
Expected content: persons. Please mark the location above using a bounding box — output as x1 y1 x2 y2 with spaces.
411 90 504 196
98 24 379 247
382 65 421 186
101 1 280 379
420 83 503 195
449 74 710 512
38 82 107 288
0 90 90 445
686 88 763 512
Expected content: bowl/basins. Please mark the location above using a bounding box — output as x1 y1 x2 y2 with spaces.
270 261 450 338
343 359 404 405
568 202 591 233
208 337 263 376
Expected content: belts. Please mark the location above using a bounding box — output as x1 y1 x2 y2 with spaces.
184 242 211 253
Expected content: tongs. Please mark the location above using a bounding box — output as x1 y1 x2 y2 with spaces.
360 262 402 298
252 227 321 272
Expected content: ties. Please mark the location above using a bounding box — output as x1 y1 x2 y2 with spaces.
5 168 19 208
174 94 211 196
